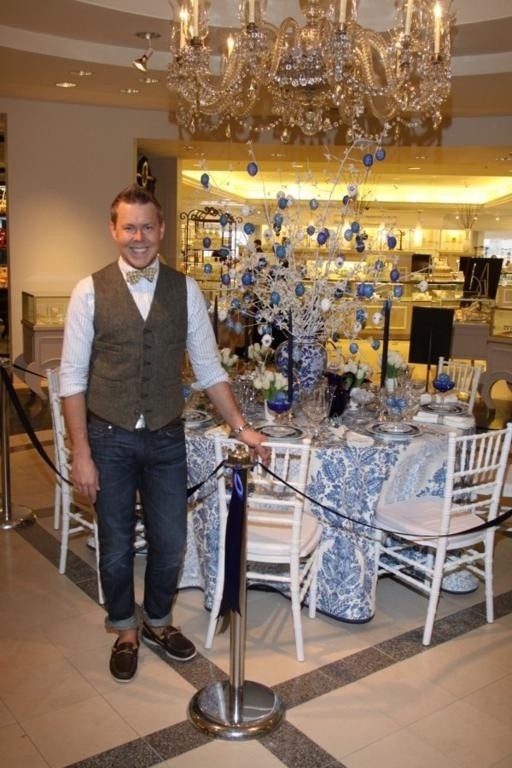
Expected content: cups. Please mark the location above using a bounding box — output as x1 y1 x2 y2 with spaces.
406 383 425 407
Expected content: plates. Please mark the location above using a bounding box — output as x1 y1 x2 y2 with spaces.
252 425 307 441
366 422 424 440
183 410 212 428
419 401 472 416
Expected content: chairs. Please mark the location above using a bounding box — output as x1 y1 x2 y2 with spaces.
201 433 325 661
436 356 483 416
40 364 144 605
374 420 510 647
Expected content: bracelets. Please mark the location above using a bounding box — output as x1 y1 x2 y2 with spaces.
232 421 249 434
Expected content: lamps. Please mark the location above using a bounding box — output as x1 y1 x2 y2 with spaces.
133 32 161 74
165 1 457 144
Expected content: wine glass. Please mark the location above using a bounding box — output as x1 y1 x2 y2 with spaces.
385 407 410 430
234 359 257 423
307 387 326 446
398 365 415 399
267 401 292 434
432 381 455 410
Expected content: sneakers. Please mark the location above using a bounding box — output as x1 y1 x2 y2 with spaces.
142 620 197 663
109 625 140 683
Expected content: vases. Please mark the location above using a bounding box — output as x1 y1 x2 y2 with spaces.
273 337 327 399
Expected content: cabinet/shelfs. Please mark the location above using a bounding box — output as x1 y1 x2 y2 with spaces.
180 207 236 299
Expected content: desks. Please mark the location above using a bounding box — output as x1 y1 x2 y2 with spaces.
178 387 475 615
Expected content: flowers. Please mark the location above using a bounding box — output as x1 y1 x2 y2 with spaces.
186 126 429 355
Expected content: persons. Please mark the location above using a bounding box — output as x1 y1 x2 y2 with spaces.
60 186 273 684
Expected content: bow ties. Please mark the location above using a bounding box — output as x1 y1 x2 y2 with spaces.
127 265 155 285
